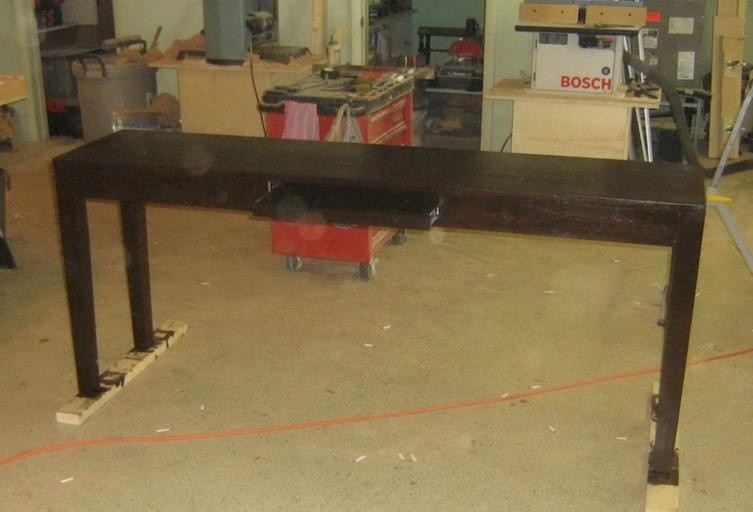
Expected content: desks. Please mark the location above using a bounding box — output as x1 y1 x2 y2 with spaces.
39 45 104 138
482 78 662 159
147 39 324 138
53 128 706 487
417 26 480 66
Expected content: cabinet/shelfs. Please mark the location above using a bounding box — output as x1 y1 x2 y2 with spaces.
260 68 416 281
